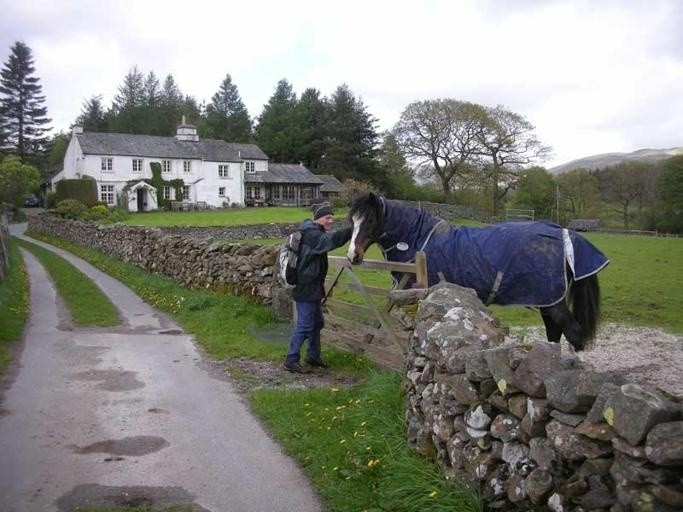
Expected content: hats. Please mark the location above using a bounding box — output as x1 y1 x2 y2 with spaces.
311 199 334 221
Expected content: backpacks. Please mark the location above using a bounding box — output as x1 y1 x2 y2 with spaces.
280 225 322 289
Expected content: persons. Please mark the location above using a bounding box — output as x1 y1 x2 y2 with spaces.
284 202 354 372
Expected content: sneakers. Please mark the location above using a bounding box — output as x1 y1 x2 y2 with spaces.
303 354 331 370
283 358 313 375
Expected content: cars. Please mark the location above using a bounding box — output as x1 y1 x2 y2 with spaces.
24 193 41 207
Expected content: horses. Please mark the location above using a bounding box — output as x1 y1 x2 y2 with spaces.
346 191 599 353
